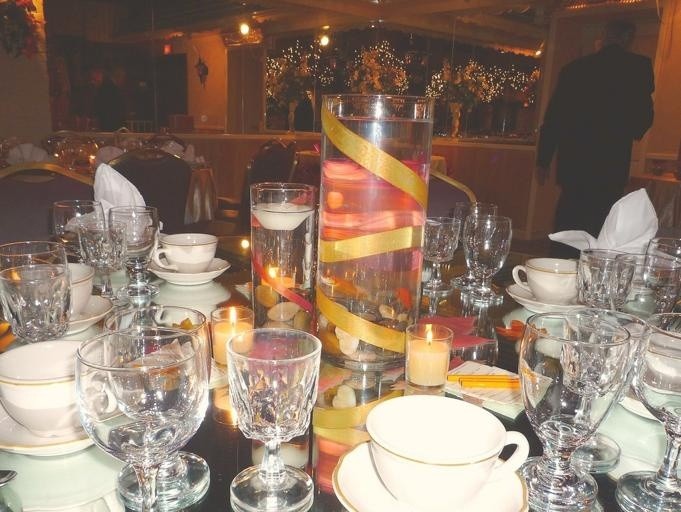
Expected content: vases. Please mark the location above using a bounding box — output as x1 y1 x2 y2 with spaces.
448 102 463 144
287 101 299 135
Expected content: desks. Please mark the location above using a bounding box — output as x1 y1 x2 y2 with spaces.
631 172 681 237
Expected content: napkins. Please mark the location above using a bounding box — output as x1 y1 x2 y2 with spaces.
6 144 48 165
95 146 124 162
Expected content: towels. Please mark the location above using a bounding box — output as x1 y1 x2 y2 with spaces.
548 187 659 253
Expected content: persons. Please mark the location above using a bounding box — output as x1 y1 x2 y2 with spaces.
87 65 126 132
535 18 655 259
294 92 313 131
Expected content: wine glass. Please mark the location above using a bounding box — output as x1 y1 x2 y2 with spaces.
105 304 211 510
616 313 681 512
459 215 512 307
449 201 500 292
75 325 210 512
107 205 161 302
547 308 653 474
225 327 322 509
78 219 130 311
517 312 631 511
423 218 463 298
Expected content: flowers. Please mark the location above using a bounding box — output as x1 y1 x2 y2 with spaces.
344 40 411 95
424 56 507 111
264 40 334 103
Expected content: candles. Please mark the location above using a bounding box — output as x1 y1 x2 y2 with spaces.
212 366 239 426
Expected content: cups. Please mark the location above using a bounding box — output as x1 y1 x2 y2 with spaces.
313 91 435 375
2 264 72 343
512 259 580 304
154 232 219 273
644 237 680 316
53 199 108 261
62 262 96 315
0 337 99 439
3 241 68 335
578 248 636 309
248 181 317 297
366 395 531 509
611 252 680 338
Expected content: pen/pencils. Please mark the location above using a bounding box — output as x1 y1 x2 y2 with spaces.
447 373 541 388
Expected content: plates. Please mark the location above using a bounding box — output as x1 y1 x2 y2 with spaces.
331 439 529 509
65 293 113 334
504 282 586 314
0 405 95 458
148 258 233 285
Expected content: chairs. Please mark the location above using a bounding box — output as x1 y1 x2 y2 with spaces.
214 137 296 231
42 128 186 153
1 160 96 251
427 170 476 221
103 148 193 234
